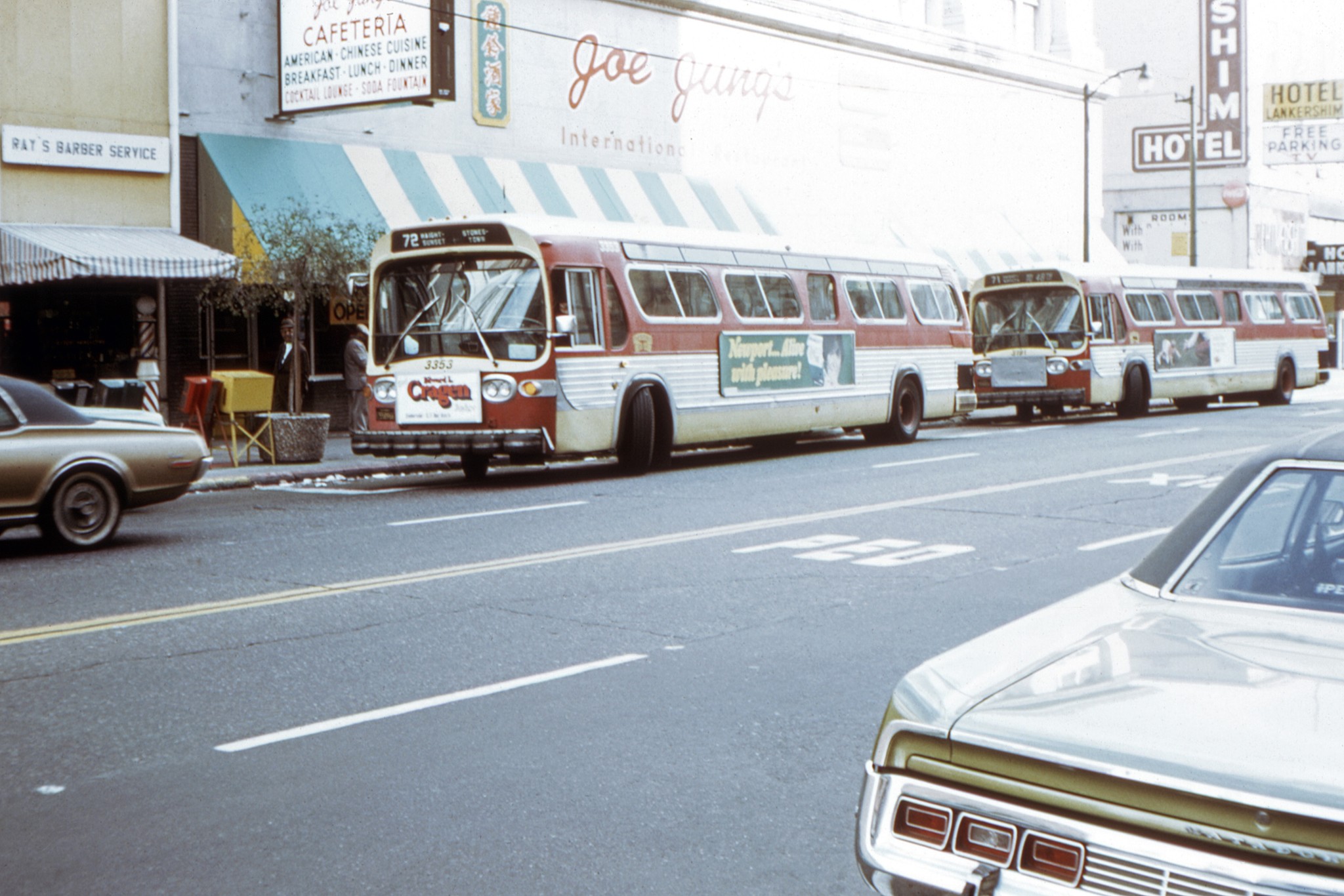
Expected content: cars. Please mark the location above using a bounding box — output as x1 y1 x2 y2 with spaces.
854 432 1344 896
0 375 213 552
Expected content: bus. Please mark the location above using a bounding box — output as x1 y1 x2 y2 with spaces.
964 265 1332 420
348 216 981 479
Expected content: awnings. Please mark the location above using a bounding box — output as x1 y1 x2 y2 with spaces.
197 132 779 284
1 222 238 285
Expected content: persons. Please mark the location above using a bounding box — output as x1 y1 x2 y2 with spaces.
344 323 369 433
275 319 310 410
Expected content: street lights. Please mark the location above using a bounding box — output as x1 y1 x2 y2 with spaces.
1084 63 1150 268
